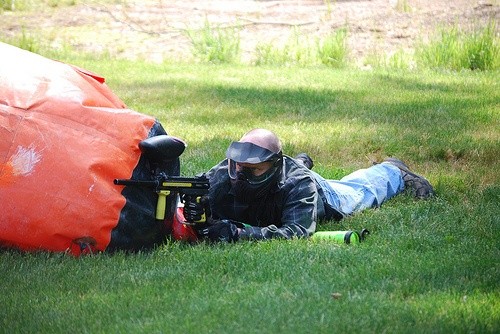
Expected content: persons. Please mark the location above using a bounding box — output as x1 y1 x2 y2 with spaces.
173 127 435 238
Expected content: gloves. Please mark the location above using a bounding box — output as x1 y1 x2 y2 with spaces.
183 196 211 226
199 218 237 244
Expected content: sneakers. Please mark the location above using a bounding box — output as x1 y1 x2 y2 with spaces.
296 153 313 169
384 157 434 200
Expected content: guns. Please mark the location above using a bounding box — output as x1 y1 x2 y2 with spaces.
114 168 212 244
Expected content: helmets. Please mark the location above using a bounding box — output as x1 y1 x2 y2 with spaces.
227 128 282 200
